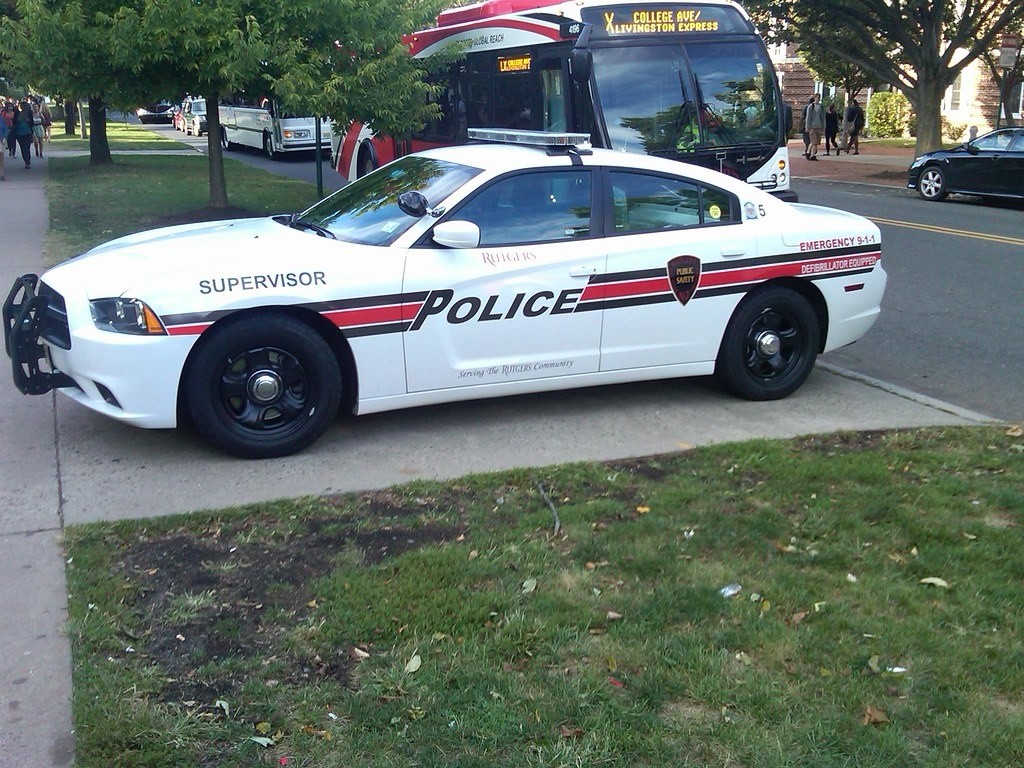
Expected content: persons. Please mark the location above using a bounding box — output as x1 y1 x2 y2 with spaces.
0 93 52 181
449 94 532 138
735 92 793 144
844 99 864 154
823 103 843 156
798 92 826 161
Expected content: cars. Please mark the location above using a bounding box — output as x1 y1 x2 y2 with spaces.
906 127 1024 205
183 99 208 138
1 127 889 462
170 101 186 130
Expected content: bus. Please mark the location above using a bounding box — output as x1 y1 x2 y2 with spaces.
137 98 175 124
216 60 332 160
330 0 801 224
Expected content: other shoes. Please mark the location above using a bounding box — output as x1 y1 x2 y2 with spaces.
823 152 830 156
24 163 30 169
40 153 42 156
835 147 840 156
36 153 38 156
12 155 16 158
8 154 11 156
802 153 807 156
805 152 810 159
844 149 849 153
810 155 818 161
852 151 859 155
5 146 8 149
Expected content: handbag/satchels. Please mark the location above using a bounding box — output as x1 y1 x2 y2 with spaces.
844 121 856 133
799 118 806 133
